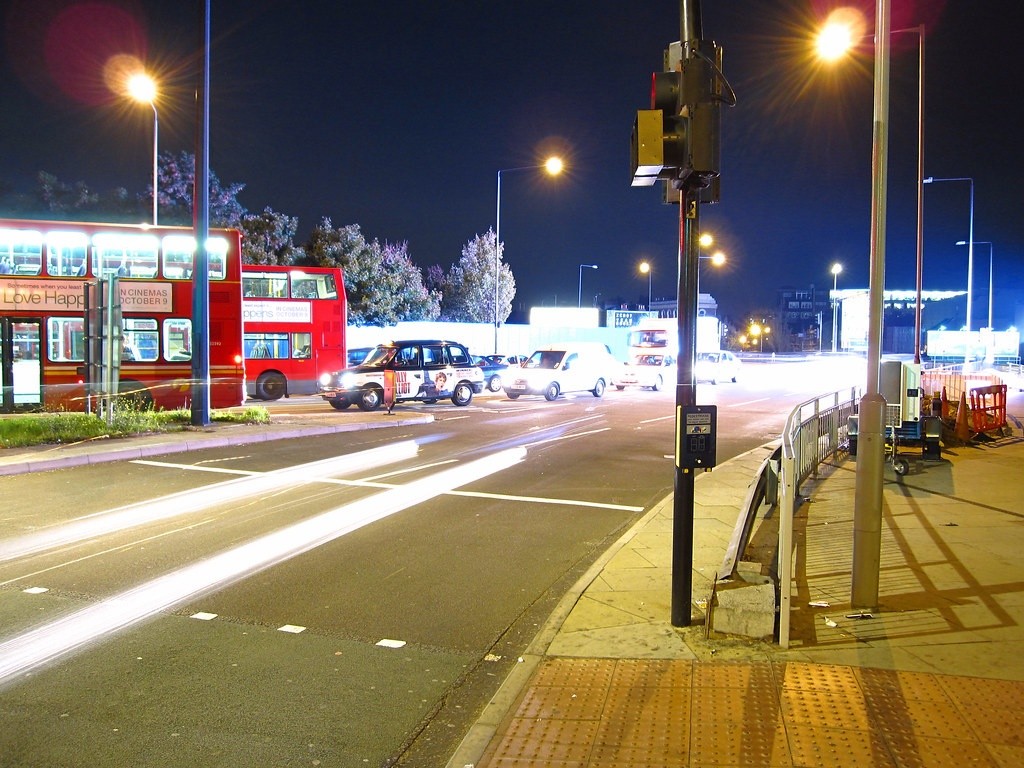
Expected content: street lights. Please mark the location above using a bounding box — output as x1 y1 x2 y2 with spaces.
925 178 975 377
494 159 562 353
955 240 995 329
832 263 843 353
578 263 598 308
640 261 651 318
130 77 163 221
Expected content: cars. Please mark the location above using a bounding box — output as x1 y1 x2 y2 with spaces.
347 346 373 367
487 354 537 370
501 344 627 401
695 350 742 386
451 355 509 393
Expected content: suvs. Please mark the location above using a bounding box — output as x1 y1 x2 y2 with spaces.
316 338 485 414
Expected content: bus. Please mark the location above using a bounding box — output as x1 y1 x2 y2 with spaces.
1 220 255 414
611 324 676 394
243 263 349 401
